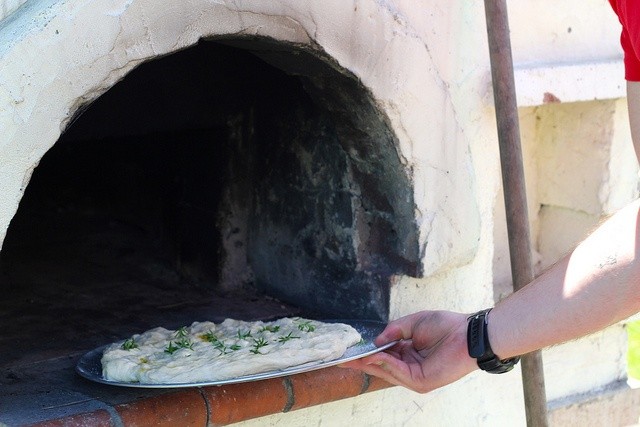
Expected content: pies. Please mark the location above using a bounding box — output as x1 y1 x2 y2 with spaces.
101 316 357 387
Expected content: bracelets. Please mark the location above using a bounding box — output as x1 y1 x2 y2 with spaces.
467 306 520 374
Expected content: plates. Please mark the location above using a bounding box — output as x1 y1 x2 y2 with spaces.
75 318 402 391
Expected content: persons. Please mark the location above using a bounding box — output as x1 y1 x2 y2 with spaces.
337 0 640 393
